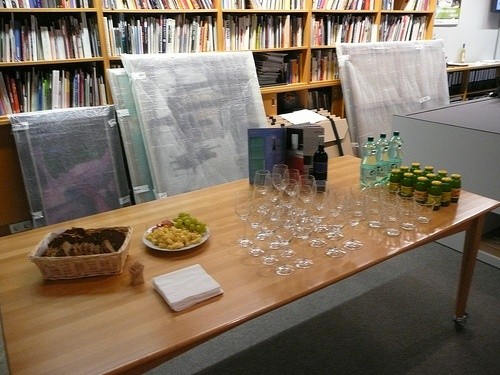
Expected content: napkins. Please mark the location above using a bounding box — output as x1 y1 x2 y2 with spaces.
152 264 223 312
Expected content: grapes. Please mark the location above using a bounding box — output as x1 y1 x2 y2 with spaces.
172 211 206 234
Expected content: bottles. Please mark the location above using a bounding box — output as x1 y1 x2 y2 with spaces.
361 131 461 212
287 149 304 186
313 143 328 192
459 44 466 63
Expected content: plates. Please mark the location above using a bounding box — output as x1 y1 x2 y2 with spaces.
142 223 210 251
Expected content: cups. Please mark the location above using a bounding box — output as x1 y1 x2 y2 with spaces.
346 184 436 236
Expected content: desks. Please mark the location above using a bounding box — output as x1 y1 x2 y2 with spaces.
0 154 500 375
393 96 500 266
446 62 500 102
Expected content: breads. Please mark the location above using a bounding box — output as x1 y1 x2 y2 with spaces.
41 239 115 257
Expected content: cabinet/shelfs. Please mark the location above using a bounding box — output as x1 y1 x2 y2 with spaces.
0 0 439 124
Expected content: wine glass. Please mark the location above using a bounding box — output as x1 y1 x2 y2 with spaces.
232 162 362 275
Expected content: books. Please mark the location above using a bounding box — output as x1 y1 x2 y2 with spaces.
0 0 430 117
